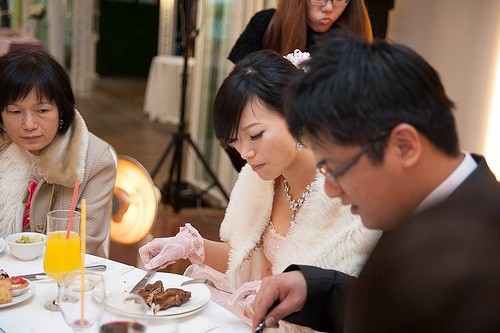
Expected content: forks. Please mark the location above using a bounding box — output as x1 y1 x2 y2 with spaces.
180 278 233 295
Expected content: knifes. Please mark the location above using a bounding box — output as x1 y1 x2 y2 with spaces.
128 269 157 295
19 264 107 281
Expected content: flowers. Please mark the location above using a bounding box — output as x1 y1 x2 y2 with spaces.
284 49 311 73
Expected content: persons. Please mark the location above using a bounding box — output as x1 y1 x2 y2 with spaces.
227 0 372 65
252 24 500 333
137 47 382 333
0 48 116 260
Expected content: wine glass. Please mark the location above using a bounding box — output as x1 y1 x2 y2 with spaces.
43 209 84 313
59 269 106 333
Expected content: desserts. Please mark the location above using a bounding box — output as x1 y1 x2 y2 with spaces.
8 276 31 297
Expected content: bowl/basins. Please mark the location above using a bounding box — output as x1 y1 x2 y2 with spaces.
5 232 46 261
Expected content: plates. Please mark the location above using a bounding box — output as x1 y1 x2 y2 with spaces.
11 277 30 296
93 272 213 320
0 282 36 309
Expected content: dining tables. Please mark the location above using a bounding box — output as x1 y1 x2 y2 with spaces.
0 240 252 333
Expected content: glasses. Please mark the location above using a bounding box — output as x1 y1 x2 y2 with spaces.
308 0 349 6
318 132 389 190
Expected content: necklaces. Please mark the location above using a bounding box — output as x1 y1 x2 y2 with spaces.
282 168 318 227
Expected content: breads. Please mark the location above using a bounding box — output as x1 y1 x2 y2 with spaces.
0 277 12 304
